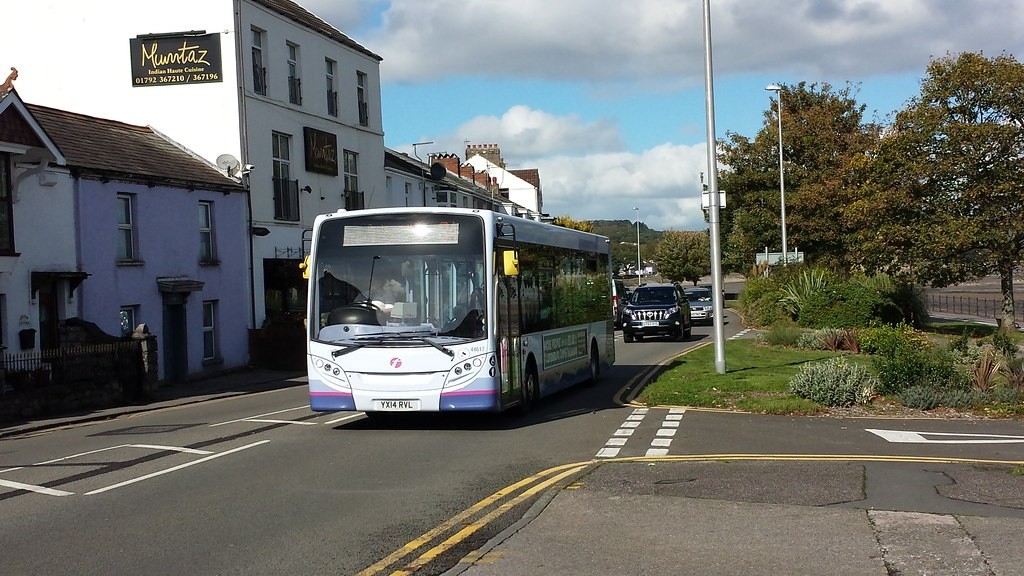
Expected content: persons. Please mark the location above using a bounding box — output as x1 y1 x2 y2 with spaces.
354 273 394 326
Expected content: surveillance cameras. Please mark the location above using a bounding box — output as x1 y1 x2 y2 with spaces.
246 164 255 170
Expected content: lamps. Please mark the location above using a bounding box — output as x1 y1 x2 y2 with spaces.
252 227 270 236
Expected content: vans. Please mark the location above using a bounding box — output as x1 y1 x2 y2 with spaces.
612 277 632 329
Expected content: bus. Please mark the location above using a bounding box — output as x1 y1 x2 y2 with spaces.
298 207 616 414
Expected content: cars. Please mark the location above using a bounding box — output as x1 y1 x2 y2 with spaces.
683 287 713 326
696 283 725 308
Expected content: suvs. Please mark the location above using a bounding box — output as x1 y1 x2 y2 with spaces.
621 280 692 344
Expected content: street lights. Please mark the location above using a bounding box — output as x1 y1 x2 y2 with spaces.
632 206 641 286
764 84 790 269
368 255 381 300
